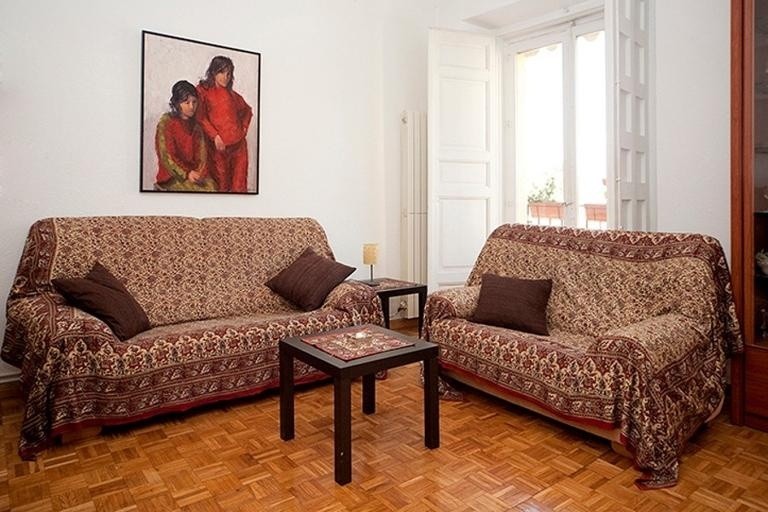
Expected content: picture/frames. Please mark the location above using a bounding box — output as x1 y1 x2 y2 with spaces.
138 29 261 196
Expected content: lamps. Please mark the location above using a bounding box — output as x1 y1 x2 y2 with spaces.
363 242 380 288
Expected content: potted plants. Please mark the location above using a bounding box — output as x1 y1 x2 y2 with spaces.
527 178 564 220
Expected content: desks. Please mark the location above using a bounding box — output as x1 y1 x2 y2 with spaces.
278 276 441 486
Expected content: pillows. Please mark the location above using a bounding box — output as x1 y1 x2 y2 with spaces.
264 242 358 313
49 262 152 343
470 271 554 337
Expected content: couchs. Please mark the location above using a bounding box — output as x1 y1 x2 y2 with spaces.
422 222 728 461
0 215 385 446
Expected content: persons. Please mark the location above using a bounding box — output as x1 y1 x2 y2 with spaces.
195 55 253 192
154 78 218 192
